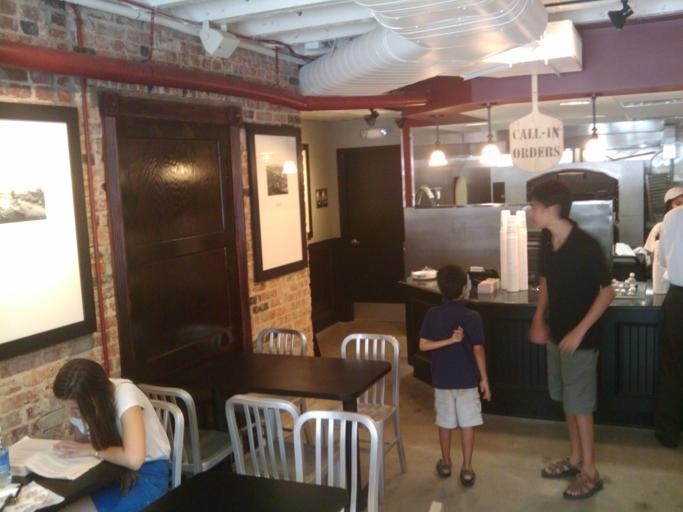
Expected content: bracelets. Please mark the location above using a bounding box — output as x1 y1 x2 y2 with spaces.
95 447 100 457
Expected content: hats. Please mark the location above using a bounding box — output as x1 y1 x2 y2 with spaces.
664 187 683 202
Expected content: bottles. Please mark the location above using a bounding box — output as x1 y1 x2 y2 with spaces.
0 441 11 489
611 273 636 296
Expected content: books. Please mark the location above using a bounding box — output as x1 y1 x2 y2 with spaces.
6 436 104 481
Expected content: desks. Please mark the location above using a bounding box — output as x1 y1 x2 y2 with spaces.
147 470 352 511
209 352 393 488
10 460 119 512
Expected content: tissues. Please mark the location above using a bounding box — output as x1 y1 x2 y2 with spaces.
469 265 498 287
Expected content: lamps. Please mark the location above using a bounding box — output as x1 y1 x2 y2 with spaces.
608 0 633 29
582 96 607 159
427 115 447 168
479 104 502 166
361 108 387 141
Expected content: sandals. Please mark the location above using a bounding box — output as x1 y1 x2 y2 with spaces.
542 456 584 478
460 470 475 486
564 469 602 499
437 459 451 476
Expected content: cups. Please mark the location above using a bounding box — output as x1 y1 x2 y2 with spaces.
500 209 529 292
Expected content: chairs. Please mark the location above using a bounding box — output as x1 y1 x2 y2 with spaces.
334 334 407 473
252 328 308 444
291 409 381 511
138 382 235 475
226 394 340 483
147 396 184 491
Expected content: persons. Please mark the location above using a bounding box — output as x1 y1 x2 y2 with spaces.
654 185 683 448
526 179 616 499
419 265 492 485
54 359 172 512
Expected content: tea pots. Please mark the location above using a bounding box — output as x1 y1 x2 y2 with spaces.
414 186 434 207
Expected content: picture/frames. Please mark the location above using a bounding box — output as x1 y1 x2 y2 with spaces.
0 98 95 360
243 114 308 283
301 144 313 242
